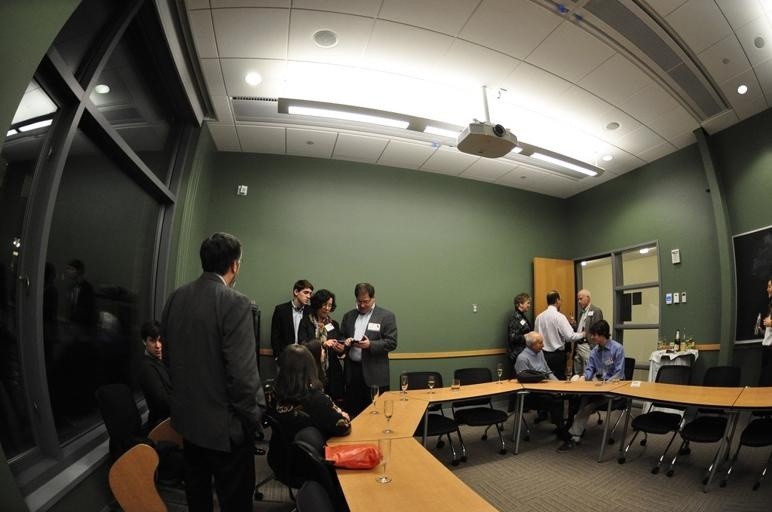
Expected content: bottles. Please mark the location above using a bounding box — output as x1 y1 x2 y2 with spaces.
754 313 762 336
674 329 681 351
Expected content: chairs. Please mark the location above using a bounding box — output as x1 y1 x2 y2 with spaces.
254 412 342 511
92 383 195 512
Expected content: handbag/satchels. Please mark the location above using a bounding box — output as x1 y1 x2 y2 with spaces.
323 443 381 469
517 370 546 382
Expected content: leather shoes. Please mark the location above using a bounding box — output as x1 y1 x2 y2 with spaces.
255 449 266 455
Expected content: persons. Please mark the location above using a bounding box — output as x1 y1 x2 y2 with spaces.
141 321 171 431
271 280 313 377
161 234 267 512
262 343 351 439
757 276 772 387
534 290 586 425
557 320 626 451
42 263 58 343
514 330 565 431
506 293 532 413
65 258 97 320
297 289 345 397
570 289 602 376
341 283 398 418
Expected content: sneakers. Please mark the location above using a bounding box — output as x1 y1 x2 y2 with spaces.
558 438 584 452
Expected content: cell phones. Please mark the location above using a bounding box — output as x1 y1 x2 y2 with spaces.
351 339 359 343
338 339 345 345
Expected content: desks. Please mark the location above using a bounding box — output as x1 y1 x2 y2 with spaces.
259 347 508 361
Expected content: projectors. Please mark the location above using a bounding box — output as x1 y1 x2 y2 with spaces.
456 123 519 158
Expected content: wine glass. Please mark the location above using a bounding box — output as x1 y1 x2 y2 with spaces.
369 385 380 415
428 375 435 394
496 364 503 384
382 400 394 434
375 438 393 483
400 376 408 402
565 367 573 383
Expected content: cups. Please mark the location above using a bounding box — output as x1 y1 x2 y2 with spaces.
451 379 460 393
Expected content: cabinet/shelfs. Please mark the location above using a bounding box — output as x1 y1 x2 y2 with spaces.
642 348 699 426
